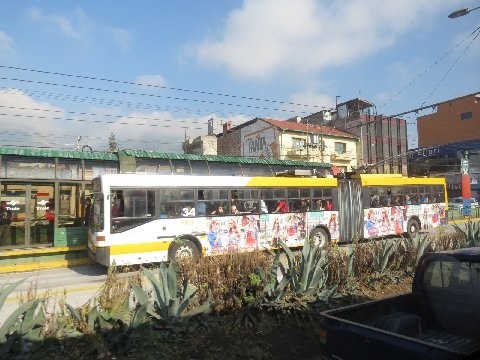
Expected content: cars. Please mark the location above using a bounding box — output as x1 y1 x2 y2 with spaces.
448 197 478 210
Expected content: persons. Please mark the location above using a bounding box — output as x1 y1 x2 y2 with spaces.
285 214 299 246
378 208 391 236
327 212 339 240
422 206 430 228
259 196 287 214
196 198 230 216
207 218 224 254
312 199 333 212
366 209 380 236
84 197 98 226
392 206 404 234
229 195 260 214
271 215 284 246
431 204 440 227
0 200 12 245
287 198 312 212
227 218 242 253
243 216 260 250
367 192 443 206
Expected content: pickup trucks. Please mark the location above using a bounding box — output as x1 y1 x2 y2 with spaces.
320 246 480 359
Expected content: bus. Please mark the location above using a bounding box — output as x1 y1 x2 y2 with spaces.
86 169 448 272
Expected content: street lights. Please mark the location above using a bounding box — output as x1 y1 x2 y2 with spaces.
448 6 480 19
